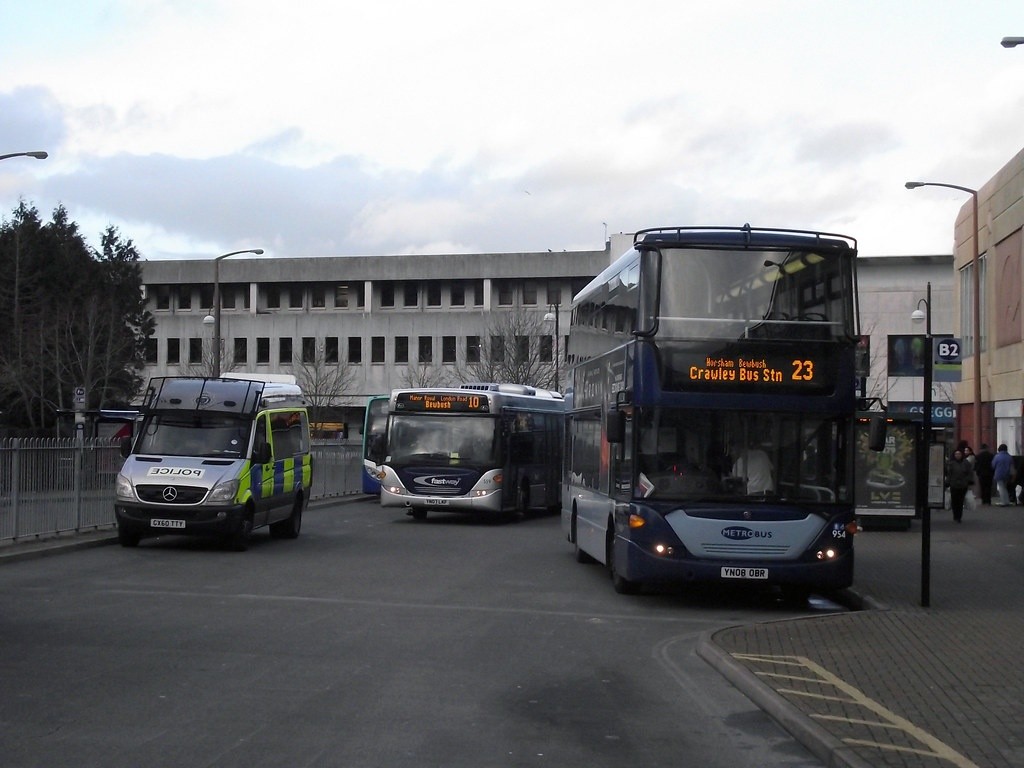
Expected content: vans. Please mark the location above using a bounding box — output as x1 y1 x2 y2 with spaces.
112 373 312 553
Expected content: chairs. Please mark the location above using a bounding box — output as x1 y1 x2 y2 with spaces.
730 312 832 340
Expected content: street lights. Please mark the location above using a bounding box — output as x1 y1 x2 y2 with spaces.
903 181 983 499
202 249 264 379
542 302 560 393
911 278 933 609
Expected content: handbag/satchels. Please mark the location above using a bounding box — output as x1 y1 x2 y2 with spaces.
963 489 977 510
944 487 952 511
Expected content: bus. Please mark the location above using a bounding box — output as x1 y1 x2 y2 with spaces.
558 225 891 601
358 394 391 494
856 409 924 534
375 382 564 523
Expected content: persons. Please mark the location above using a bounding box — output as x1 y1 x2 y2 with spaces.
731 433 788 498
339 430 343 440
634 434 672 479
944 439 1023 524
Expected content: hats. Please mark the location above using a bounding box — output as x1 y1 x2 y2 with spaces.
980 444 988 449
998 444 1008 451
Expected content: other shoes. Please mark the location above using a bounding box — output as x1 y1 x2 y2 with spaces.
995 503 1005 507
